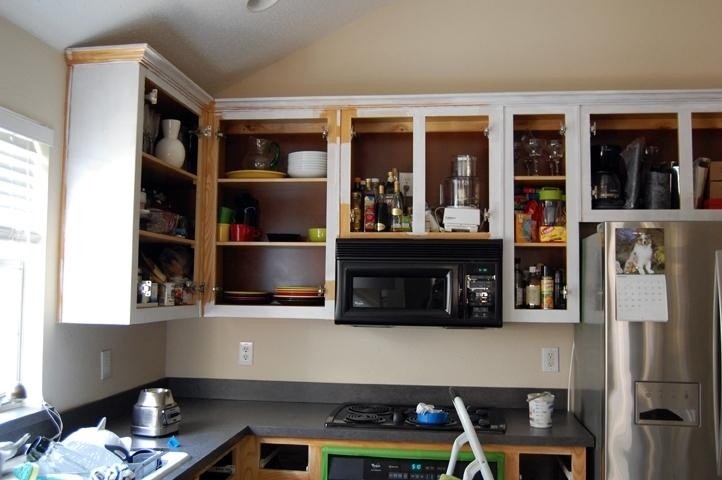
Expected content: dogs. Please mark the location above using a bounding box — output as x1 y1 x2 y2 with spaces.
623 233 656 275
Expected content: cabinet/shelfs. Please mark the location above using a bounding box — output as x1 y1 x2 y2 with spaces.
504 89 579 322
60 45 214 327
578 90 722 223
338 96 510 239
201 93 341 321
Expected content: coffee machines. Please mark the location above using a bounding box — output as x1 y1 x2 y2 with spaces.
592 145 628 208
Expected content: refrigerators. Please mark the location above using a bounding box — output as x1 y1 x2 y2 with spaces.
574 221 722 480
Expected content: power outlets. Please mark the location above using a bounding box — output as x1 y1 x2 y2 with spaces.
541 347 559 374
236 340 254 365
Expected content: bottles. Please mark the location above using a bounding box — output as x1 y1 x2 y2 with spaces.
138 250 196 306
514 256 566 309
514 187 565 243
352 167 412 231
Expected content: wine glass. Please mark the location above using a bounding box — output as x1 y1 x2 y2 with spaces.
515 138 563 176
145 109 159 158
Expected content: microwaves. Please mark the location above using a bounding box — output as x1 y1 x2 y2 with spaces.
334 237 503 327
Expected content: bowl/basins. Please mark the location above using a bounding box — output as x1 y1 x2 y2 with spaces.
142 210 180 234
176 216 195 238
221 138 280 168
416 412 450 424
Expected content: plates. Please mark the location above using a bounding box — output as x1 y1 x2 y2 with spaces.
286 149 328 179
266 232 301 242
221 285 324 307
223 169 285 178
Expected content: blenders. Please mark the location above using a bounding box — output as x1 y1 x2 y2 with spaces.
439 155 481 233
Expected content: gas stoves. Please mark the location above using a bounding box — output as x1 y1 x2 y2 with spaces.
322 401 507 480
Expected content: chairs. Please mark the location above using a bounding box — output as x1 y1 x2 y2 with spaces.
434 399 496 480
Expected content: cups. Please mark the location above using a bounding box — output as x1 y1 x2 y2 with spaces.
307 227 328 242
217 202 261 241
632 146 680 209
